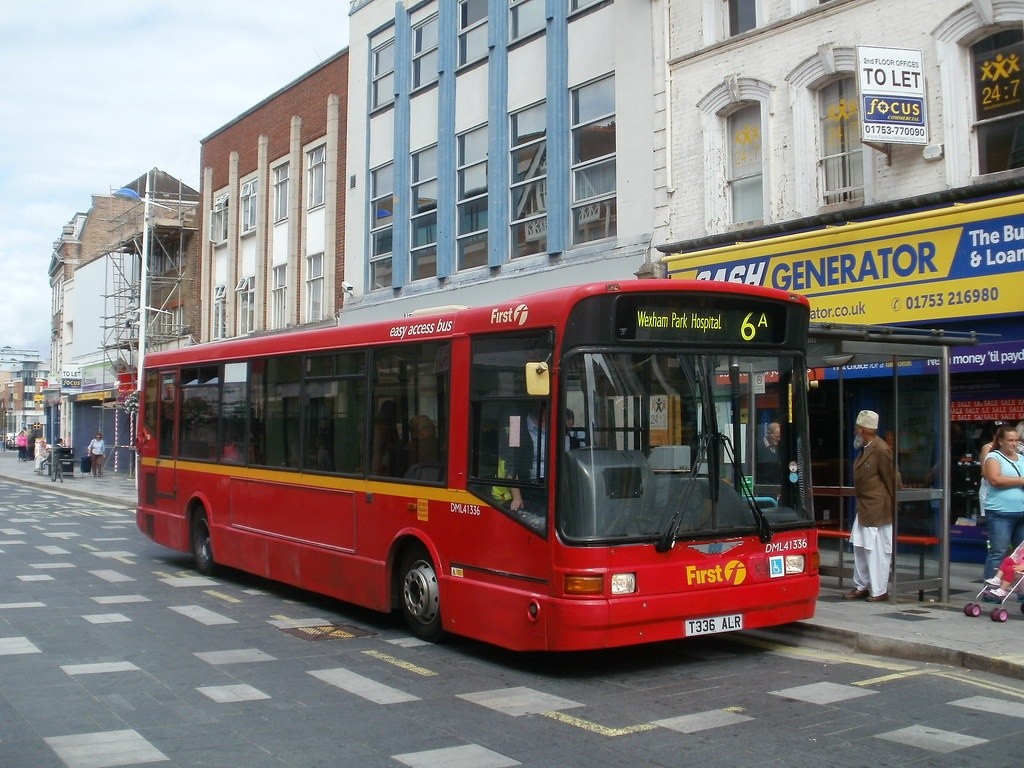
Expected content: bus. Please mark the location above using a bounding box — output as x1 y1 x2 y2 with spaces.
132 278 822 659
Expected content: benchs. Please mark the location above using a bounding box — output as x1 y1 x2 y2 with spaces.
816 528 942 601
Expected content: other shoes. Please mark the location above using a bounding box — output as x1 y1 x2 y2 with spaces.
990 588 1006 597
842 588 869 600
865 592 889 602
982 592 1005 604
1017 588 1024 600
985 576 1002 586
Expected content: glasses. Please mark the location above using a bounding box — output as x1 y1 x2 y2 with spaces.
994 420 1010 426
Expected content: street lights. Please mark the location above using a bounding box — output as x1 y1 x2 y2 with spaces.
112 169 178 394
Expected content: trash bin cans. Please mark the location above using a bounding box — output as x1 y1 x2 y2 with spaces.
48 447 74 477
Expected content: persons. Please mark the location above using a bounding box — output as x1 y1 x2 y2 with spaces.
979 419 1024 604
17 426 42 461
843 410 901 601
985 546 1024 597
353 400 442 478
506 398 580 512
55 438 63 463
755 422 785 507
88 433 105 477
33 441 51 472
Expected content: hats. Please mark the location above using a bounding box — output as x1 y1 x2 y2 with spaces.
856 410 879 429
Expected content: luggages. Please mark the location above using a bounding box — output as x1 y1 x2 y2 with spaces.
81 455 91 474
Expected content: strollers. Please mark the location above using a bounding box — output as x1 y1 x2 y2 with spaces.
964 537 1024 623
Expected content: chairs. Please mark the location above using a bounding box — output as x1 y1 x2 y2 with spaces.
405 462 448 484
480 427 508 453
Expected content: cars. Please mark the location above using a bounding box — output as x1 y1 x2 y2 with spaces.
6 435 18 451
